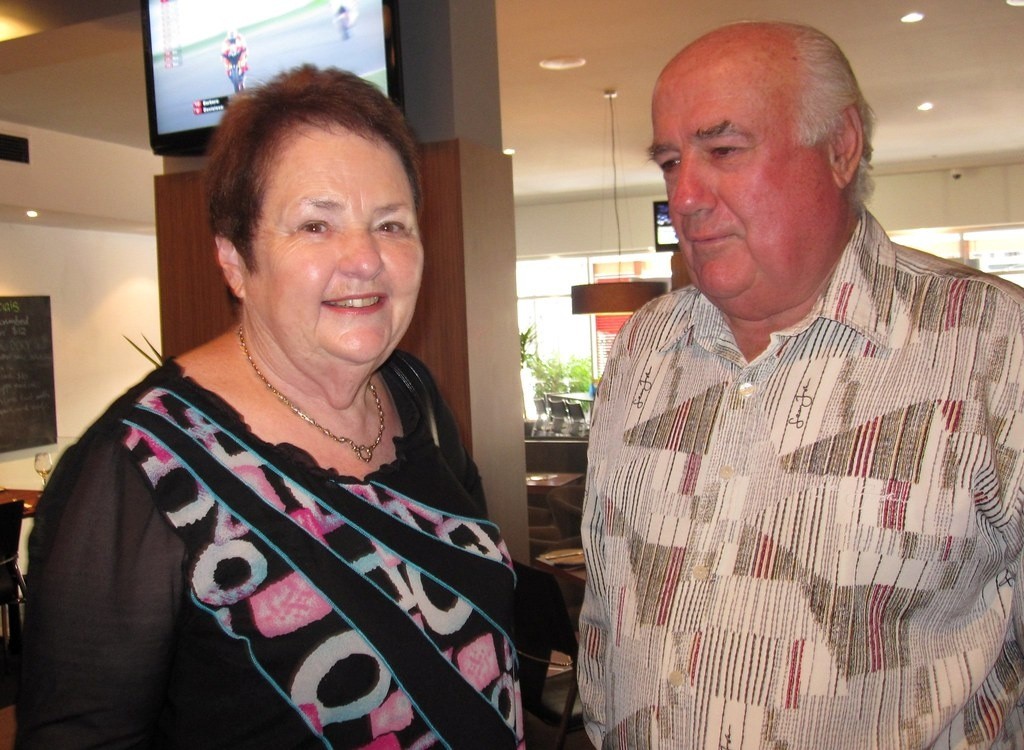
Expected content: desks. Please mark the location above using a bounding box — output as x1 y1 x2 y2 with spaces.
0 489 44 517
535 547 586 641
526 470 585 495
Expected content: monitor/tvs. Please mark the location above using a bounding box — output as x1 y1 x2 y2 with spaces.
654 201 681 252
141 0 403 156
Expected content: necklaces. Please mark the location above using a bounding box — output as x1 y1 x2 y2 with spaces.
237 327 385 463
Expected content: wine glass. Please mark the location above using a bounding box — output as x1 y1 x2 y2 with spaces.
34 453 53 497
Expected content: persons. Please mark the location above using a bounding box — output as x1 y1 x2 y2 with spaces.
577 20 1024 750
16 62 525 749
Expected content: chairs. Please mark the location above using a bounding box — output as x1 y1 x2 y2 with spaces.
0 499 27 654
546 485 585 540
512 560 584 750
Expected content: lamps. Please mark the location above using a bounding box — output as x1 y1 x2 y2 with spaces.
570 90 669 315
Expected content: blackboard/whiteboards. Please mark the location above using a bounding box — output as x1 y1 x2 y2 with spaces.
0 296 58 464
